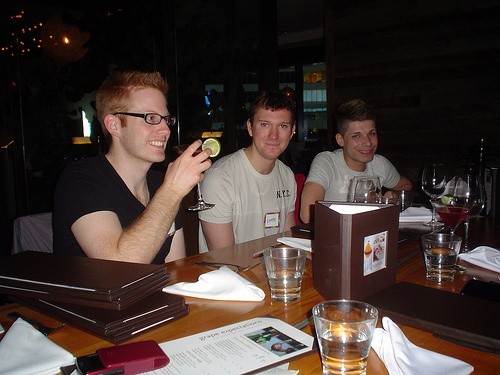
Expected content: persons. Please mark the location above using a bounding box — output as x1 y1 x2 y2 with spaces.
52 70 212 264
255 332 303 353
199 91 297 252
300 99 412 224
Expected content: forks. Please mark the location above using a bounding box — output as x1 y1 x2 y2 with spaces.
7 311 68 336
193 261 261 272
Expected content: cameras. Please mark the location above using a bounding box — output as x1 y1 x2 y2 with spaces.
75 340 170 375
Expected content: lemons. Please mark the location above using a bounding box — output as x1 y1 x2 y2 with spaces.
441 194 454 204
202 138 220 157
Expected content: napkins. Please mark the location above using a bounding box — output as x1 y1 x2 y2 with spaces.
163 266 266 302
372 317 474 375
0 318 76 375
399 206 433 224
458 246 500 273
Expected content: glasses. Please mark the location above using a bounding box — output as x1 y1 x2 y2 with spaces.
113 111 176 127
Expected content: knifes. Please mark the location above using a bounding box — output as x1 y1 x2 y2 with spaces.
252 243 283 258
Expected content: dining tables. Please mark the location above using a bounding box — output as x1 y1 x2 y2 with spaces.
0 230 500 375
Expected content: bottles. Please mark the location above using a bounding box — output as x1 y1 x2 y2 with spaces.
474 137 487 215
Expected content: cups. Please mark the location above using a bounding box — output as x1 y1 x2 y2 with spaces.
312 299 379 375
348 176 412 213
421 233 464 282
263 248 307 302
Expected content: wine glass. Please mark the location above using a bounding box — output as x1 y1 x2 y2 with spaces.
433 207 469 236
172 143 216 210
421 160 447 228
453 173 486 254
